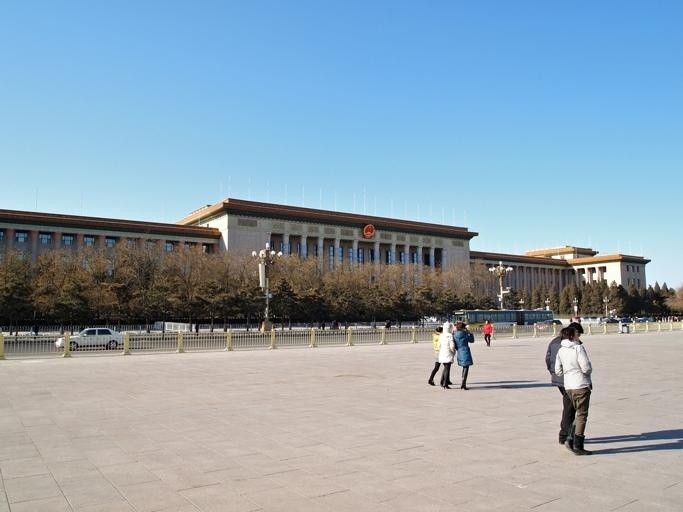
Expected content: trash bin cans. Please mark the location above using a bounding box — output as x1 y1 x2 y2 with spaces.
622 324 629 333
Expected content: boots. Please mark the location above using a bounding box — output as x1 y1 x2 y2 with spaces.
559 432 593 455
461 375 469 391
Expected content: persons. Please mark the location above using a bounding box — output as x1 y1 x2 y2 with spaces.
32 324 39 336
482 320 492 347
554 327 592 456
453 321 474 390
437 322 456 389
546 322 584 445
428 327 452 386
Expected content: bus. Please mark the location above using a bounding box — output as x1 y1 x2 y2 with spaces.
450 309 554 331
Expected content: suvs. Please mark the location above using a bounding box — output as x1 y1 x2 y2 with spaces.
537 319 563 331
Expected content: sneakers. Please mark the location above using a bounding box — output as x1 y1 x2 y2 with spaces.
428 380 436 386
486 342 491 347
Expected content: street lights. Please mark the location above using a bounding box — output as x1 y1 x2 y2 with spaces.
602 296 609 316
543 297 550 311
518 298 524 309
489 261 513 309
249 243 284 331
573 297 579 318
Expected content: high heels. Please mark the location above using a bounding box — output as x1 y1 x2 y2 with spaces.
440 380 453 390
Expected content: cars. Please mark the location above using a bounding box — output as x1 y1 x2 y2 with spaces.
581 314 680 326
419 315 447 325
53 325 124 352
